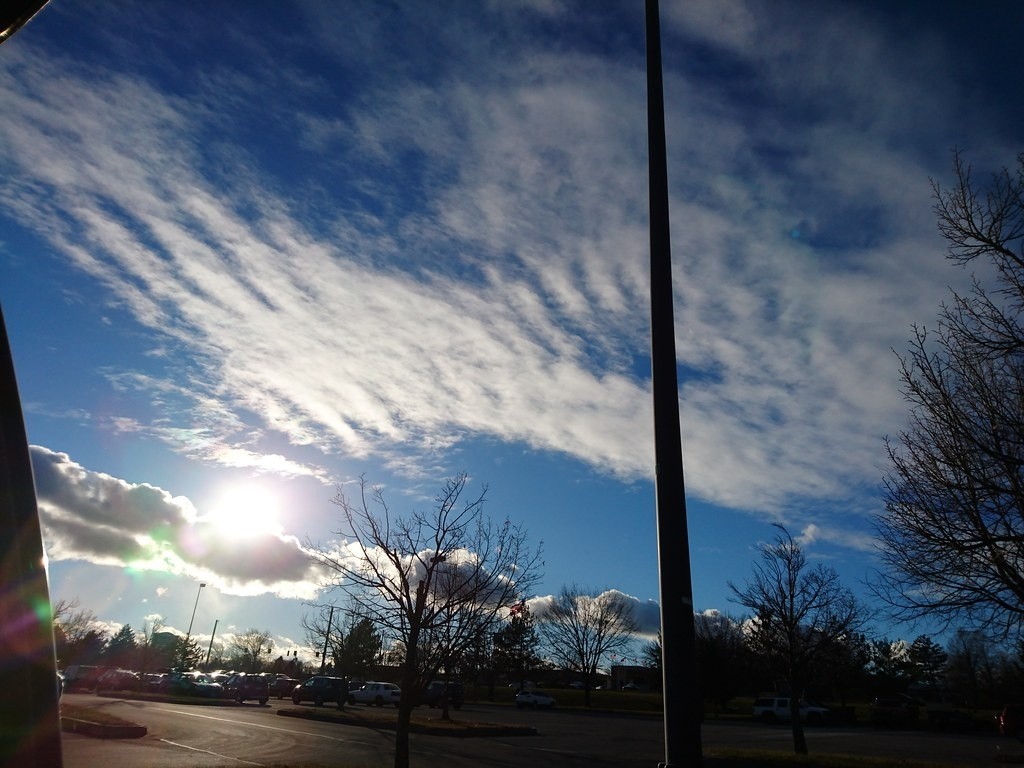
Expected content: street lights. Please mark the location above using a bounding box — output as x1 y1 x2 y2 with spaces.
180 583 207 672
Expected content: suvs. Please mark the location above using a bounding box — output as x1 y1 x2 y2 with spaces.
515 688 558 711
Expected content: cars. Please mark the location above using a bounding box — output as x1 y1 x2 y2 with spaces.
58 661 462 711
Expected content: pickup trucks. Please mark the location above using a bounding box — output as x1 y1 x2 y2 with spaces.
751 696 832 727
